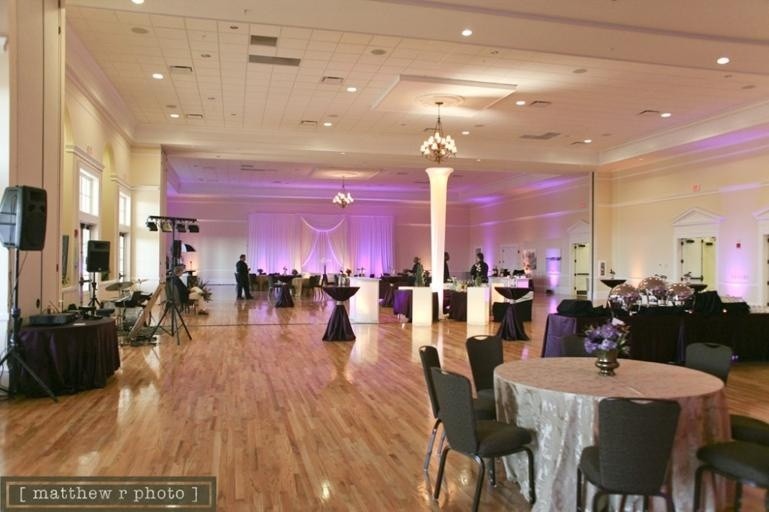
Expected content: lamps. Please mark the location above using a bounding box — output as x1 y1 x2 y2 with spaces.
333 176 355 208
146 216 199 232
420 102 457 164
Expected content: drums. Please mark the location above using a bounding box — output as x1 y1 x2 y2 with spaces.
123 307 151 329
102 300 120 318
110 296 126 317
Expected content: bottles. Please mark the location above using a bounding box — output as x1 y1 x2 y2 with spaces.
446 274 482 290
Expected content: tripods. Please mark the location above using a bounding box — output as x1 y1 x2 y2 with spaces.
87 272 102 307
150 221 192 344
0 250 59 403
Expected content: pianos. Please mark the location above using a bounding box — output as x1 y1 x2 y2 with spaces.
115 291 152 308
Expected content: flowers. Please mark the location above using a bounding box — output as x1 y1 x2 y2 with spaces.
583 322 632 353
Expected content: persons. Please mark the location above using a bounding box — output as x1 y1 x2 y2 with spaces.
404 256 425 286
171 265 209 315
444 252 452 283
470 253 488 284
237 255 253 300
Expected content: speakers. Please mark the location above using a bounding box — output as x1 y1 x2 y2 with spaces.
87 240 110 272
1 184 48 250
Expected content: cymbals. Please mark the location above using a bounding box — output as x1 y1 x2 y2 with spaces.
105 281 133 290
134 278 148 283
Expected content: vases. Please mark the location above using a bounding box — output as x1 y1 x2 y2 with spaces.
594 349 623 376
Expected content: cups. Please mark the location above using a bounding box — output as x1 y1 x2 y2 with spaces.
658 298 684 307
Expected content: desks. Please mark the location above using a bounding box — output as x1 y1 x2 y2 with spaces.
10 317 117 397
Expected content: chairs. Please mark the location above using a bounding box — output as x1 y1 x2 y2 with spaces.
731 414 769 445
466 335 504 401
693 439 769 512
419 346 501 472
578 397 682 512
684 342 733 383
234 269 769 363
432 367 534 512
